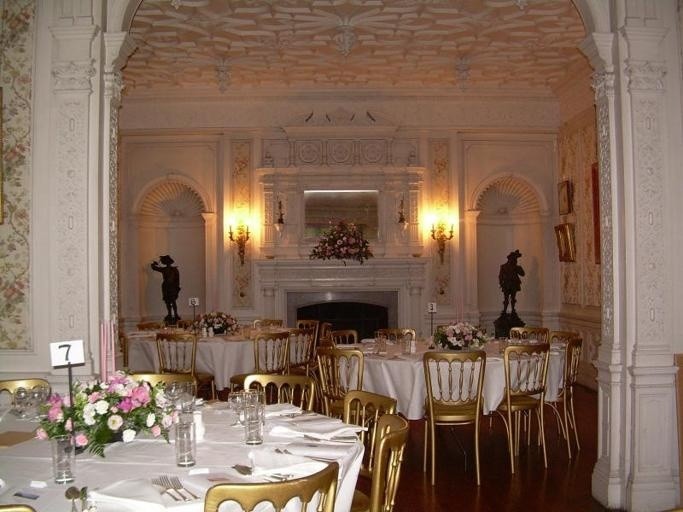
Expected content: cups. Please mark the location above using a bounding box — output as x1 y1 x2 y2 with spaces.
248 455 268 481
375 334 415 358
228 389 264 446
498 337 507 356
233 322 276 338
175 383 196 467
51 435 75 484
202 327 213 337
158 319 194 336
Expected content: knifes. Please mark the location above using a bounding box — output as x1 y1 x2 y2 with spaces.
80 487 89 512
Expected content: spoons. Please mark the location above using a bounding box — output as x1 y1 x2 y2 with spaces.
65 486 80 512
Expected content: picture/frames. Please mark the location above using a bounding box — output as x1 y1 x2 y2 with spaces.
556 181 572 215
553 224 576 262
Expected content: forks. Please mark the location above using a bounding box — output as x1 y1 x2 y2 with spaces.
152 473 200 502
302 435 357 444
274 449 337 463
231 464 294 482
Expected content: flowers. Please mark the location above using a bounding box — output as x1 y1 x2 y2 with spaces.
308 217 373 266
39 367 177 455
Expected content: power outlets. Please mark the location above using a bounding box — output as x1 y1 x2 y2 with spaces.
187 297 199 306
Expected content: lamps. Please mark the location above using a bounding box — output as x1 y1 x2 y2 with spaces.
430 211 457 264
393 192 411 239
226 209 256 264
274 199 286 238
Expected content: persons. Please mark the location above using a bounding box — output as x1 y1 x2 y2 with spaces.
151 255 181 321
496 250 525 314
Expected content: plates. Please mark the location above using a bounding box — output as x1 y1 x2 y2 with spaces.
507 339 537 344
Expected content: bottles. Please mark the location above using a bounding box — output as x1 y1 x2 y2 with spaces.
191 411 205 443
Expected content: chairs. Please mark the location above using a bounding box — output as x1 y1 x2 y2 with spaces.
204 462 340 511
2 504 32 511
0 378 52 406
350 414 409 511
98 312 586 486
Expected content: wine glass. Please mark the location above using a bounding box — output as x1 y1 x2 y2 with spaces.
15 390 47 421
166 384 182 405
